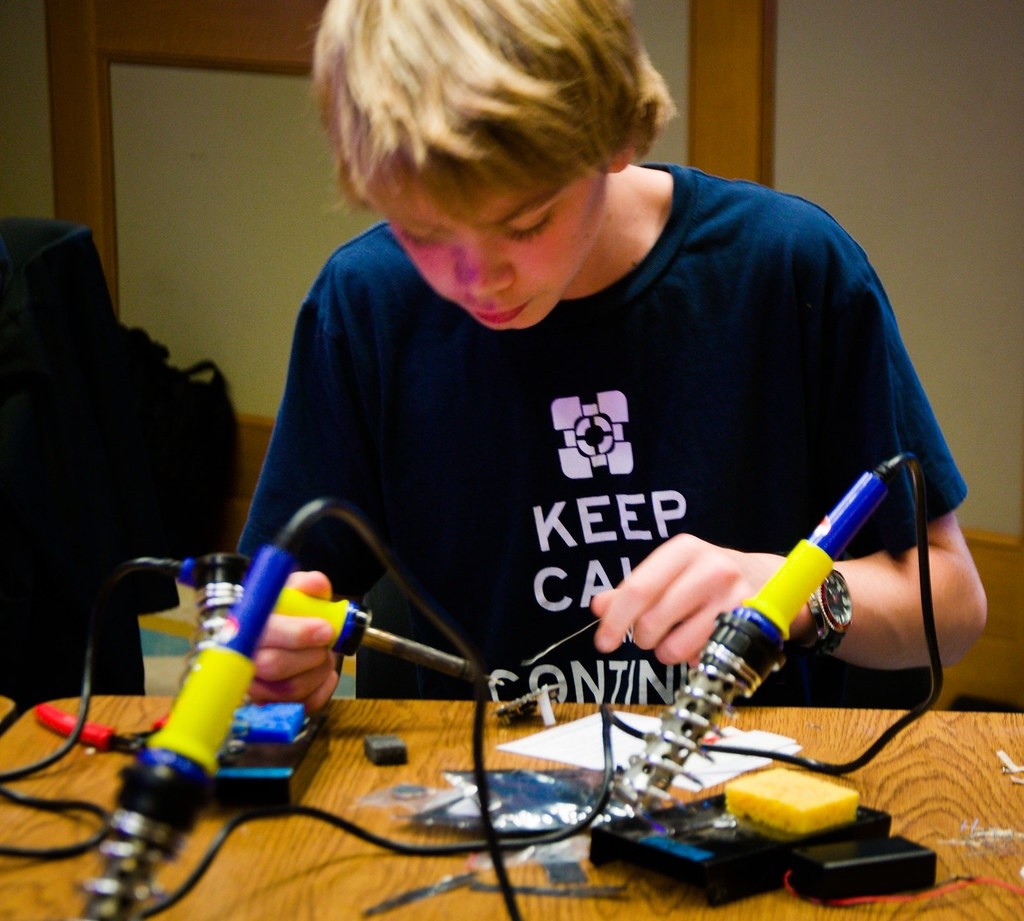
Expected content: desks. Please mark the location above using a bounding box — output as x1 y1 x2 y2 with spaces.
0 695 1024 921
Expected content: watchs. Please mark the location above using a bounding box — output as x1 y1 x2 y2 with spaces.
801 569 853 651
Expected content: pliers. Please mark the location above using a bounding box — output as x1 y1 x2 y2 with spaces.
35 700 170 756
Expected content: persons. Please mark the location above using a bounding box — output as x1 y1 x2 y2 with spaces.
232 0 988 717
